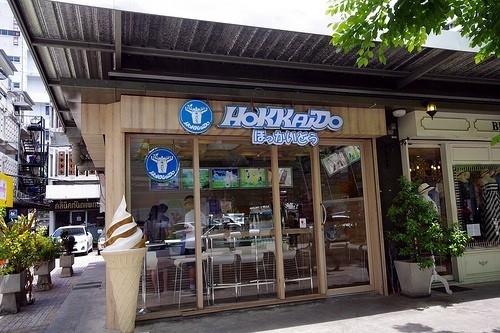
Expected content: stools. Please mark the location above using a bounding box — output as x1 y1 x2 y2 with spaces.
348 238 369 281
143 233 315 309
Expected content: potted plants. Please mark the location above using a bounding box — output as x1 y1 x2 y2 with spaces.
59 230 77 267
32 231 58 276
386 176 474 298
0 207 31 294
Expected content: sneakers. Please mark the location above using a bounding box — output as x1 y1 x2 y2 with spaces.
182 288 196 295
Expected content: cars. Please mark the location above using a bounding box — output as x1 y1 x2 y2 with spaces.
96 227 106 255
49 225 93 259
167 205 365 242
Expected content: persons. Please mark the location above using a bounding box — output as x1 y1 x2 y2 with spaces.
418 169 500 247
145 203 303 295
183 195 207 291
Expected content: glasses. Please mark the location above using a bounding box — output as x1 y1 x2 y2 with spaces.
183 201 189 205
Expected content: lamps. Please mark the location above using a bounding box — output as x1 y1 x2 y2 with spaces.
72 143 88 166
243 89 266 112
424 101 440 121
75 157 94 173
392 109 406 117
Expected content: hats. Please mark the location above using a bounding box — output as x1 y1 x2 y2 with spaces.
456 170 471 181
417 183 435 194
480 168 489 178
